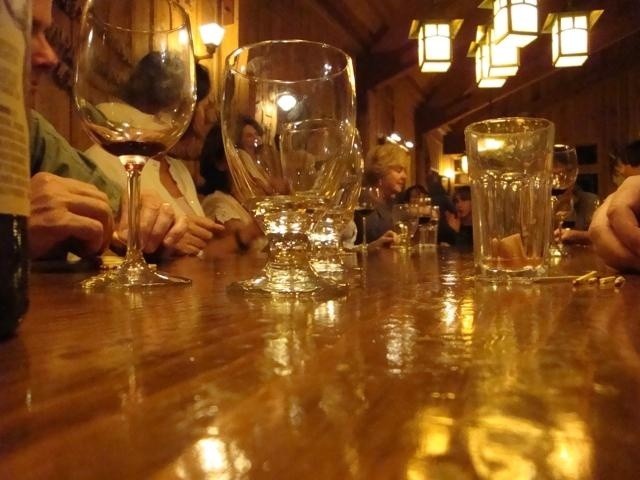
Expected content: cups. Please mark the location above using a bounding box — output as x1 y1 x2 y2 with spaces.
464 115 556 280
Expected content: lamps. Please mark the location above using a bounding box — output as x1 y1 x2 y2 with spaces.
408 0 604 87
196 22 224 63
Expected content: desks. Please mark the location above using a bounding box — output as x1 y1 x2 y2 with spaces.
0 244 640 480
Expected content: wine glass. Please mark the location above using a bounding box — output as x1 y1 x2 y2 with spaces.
550 143 579 258
391 194 439 252
221 39 379 299
67 1 199 292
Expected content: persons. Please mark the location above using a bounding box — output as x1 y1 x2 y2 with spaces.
193 110 639 280
83 47 290 261
22 0 191 266
